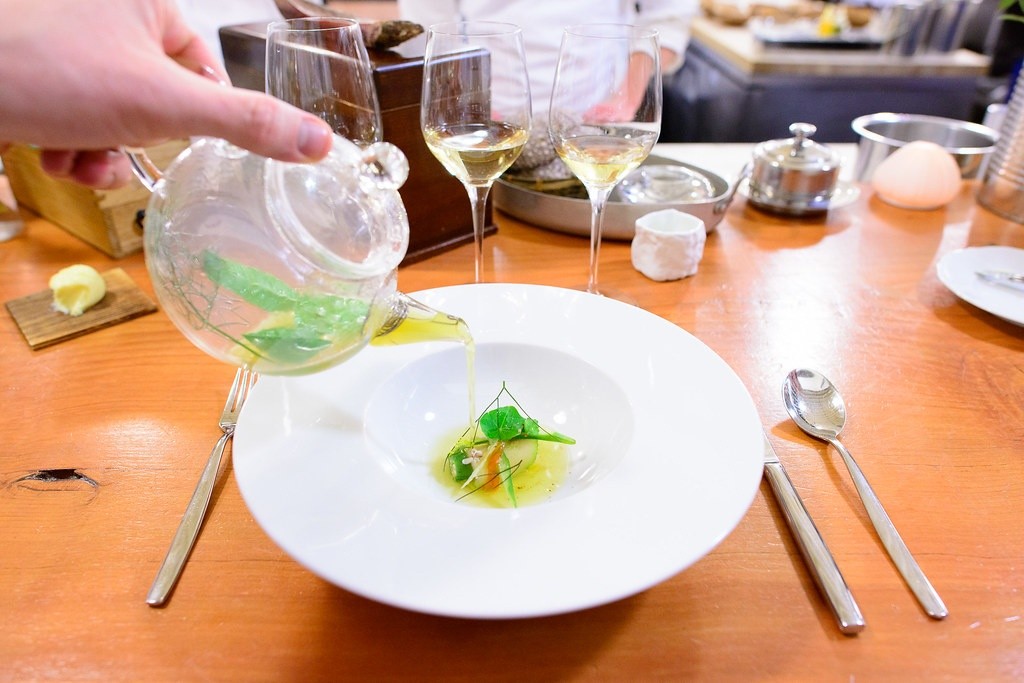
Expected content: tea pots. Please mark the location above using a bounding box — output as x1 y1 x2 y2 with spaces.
120 58 471 379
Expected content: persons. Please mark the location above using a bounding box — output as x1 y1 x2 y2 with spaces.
320 0 700 126
0 0 332 189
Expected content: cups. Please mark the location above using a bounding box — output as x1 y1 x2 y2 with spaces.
749 120 842 202
875 4 925 55
975 66 1024 226
265 15 386 152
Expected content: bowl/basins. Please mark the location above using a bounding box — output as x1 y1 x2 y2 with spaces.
850 111 1001 180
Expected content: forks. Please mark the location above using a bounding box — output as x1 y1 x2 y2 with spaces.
143 366 265 606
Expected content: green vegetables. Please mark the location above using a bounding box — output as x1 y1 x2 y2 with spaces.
449 405 577 509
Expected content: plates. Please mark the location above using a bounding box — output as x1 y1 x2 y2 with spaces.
229 281 766 619
935 244 1024 326
733 171 861 217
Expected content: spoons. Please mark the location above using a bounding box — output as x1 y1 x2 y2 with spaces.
780 366 949 623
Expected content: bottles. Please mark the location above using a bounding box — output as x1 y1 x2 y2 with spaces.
617 163 714 204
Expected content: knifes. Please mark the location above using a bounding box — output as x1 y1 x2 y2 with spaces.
763 432 867 632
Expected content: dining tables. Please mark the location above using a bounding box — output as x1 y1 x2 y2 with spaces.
657 17 993 143
0 142 1024 683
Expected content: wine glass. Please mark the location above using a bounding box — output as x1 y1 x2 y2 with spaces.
418 20 534 283
546 22 664 303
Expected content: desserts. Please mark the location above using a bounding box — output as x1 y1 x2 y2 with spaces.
48 263 105 317
